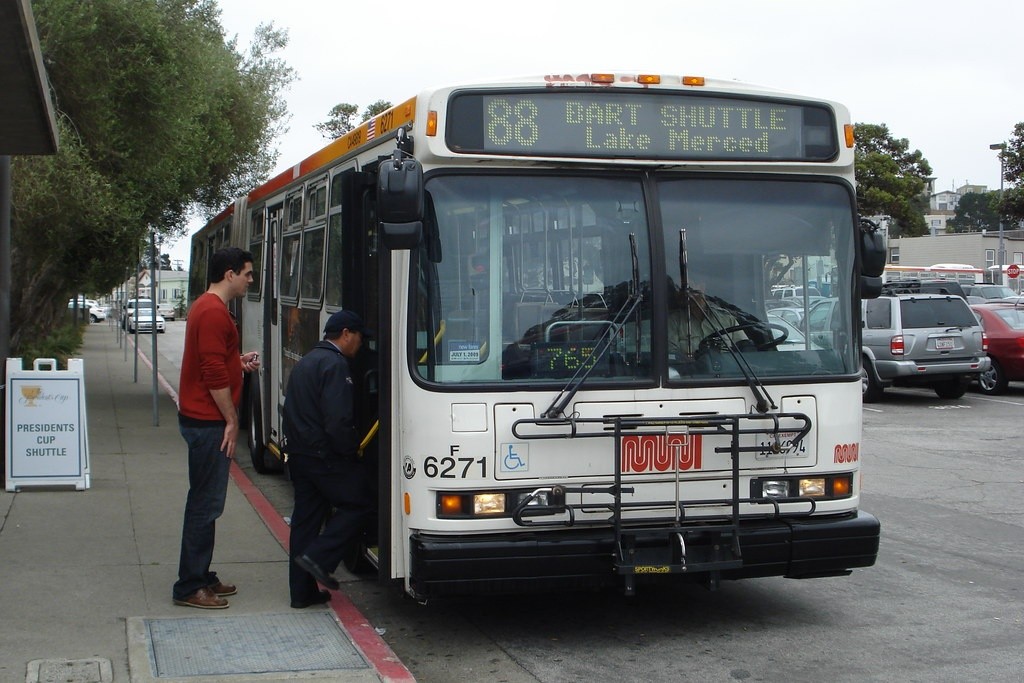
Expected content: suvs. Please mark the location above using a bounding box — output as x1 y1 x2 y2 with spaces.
122 299 159 324
770 285 822 306
880 279 969 303
797 286 990 404
67 298 105 323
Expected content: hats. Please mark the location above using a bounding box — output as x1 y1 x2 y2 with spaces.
325 309 370 334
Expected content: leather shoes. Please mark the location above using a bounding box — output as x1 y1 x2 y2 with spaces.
173 585 230 609
207 581 238 595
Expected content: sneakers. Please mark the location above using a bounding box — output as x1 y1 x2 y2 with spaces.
291 589 330 608
293 553 339 590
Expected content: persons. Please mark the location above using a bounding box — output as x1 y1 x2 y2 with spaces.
667 265 750 357
281 311 376 608
172 247 259 609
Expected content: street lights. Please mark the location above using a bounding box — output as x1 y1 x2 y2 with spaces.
990 143 1005 284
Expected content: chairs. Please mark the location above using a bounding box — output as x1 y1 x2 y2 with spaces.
625 319 651 368
446 289 609 351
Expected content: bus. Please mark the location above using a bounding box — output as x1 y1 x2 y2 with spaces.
882 263 983 295
187 72 882 606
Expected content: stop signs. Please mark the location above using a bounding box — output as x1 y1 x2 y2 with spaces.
1007 265 1020 278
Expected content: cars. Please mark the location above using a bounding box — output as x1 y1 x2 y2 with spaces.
767 307 813 329
766 311 823 352
128 309 166 333
967 302 1024 396
